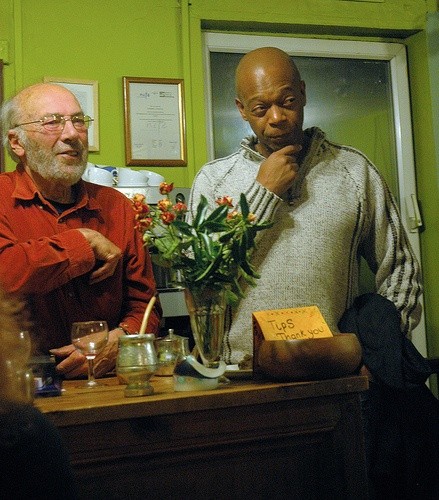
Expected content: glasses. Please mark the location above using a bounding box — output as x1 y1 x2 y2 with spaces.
9 114 94 130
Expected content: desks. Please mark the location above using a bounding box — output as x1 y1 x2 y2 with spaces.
31 363 369 500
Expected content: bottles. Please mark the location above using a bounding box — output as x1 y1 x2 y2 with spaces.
154 328 190 392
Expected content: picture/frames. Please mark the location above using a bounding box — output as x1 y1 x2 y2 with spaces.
44 76 100 152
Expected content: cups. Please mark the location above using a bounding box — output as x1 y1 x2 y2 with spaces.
80 161 167 200
115 334 156 397
0 328 34 408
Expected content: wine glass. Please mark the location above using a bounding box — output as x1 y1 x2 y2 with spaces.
71 321 109 391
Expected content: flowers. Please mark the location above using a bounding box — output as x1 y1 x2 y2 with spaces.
132 180 275 302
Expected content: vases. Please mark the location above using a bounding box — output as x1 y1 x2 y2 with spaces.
184 288 227 367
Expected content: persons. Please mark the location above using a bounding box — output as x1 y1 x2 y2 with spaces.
184 46 425 385
0 84 164 378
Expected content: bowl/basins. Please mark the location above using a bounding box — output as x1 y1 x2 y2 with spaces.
259 332 360 382
